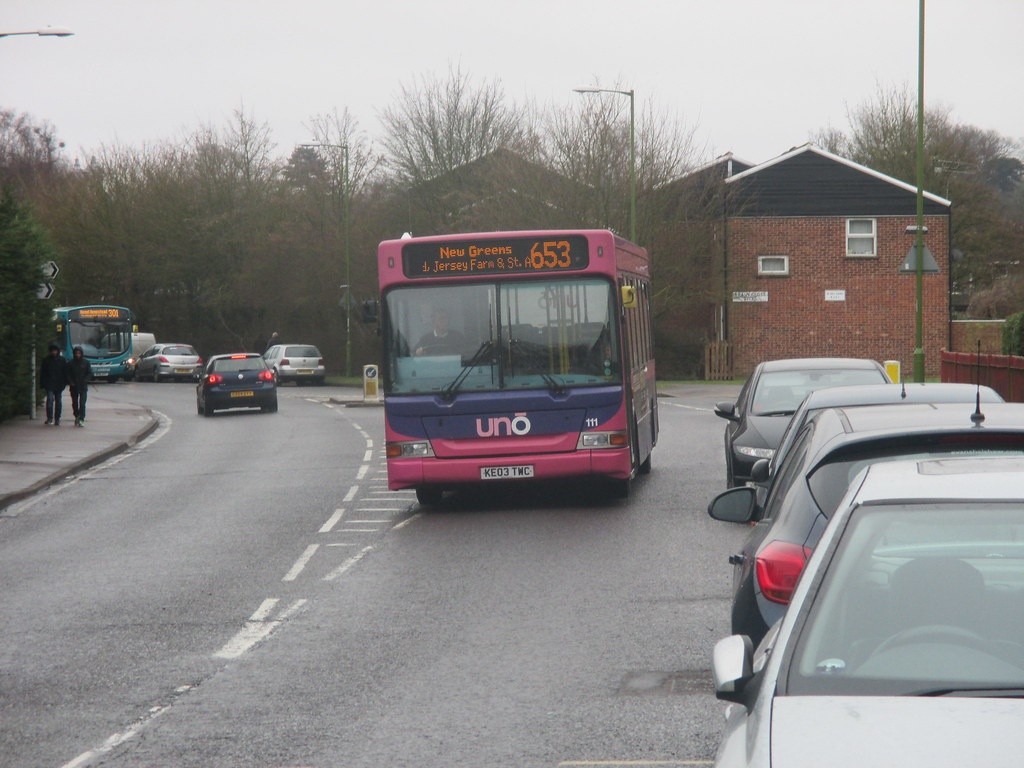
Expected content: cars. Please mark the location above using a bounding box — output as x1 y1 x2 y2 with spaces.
713 358 896 492
136 343 204 383
707 402 1023 654
192 351 279 417
710 448 1024 768
742 382 1008 529
261 344 327 386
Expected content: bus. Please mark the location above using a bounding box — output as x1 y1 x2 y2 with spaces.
49 305 136 383
377 228 661 508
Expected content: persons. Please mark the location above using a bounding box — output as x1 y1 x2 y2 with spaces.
414 309 466 356
65 346 92 426
39 343 66 425
590 343 617 375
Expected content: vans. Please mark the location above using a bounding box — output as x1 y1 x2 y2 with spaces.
99 332 156 359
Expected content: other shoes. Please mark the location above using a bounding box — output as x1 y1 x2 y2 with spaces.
45 416 53 425
55 418 60 426
75 417 84 427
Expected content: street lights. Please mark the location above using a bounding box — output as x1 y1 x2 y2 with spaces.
574 89 637 244
301 143 355 378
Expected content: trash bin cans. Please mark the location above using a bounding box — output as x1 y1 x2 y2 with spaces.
883 360 901 384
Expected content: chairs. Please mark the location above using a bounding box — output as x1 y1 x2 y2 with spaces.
847 556 996 679
498 323 609 376
763 386 795 408
